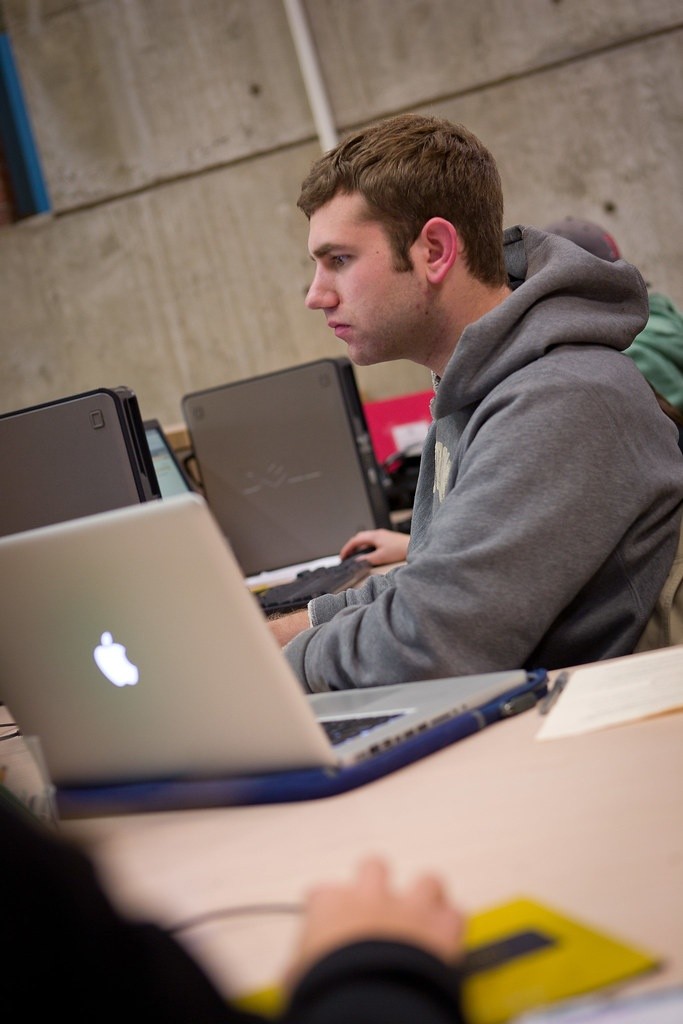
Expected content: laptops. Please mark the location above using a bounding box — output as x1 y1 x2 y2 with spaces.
0 490 549 817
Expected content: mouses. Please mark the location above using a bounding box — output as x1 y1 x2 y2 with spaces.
343 543 376 563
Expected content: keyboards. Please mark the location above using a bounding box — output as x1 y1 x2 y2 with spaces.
259 557 370 616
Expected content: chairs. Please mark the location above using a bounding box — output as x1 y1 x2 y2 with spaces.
633 506 683 654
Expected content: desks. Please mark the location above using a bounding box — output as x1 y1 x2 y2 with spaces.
0 507 413 723
0 642 683 1024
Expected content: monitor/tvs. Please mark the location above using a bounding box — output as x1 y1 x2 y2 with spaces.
141 418 193 500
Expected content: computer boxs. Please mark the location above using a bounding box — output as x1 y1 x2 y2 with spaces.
182 353 394 578
0 385 162 537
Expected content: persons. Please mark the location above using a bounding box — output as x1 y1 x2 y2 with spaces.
0 782 470 1024
546 216 683 410
269 114 683 693
